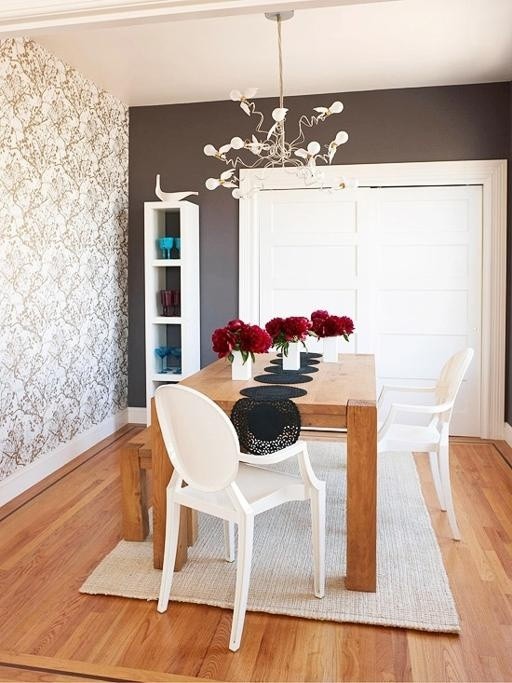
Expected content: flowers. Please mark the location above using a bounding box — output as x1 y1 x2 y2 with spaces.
212 310 355 367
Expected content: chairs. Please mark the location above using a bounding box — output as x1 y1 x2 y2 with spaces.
344 346 475 541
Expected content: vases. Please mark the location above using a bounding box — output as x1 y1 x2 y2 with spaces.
155 383 327 653
282 340 300 372
230 350 253 380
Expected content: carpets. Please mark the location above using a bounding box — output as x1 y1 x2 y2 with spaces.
78 440 463 635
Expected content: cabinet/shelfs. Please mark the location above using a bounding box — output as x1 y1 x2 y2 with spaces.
144 200 200 426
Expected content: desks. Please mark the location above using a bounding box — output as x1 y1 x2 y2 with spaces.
149 351 378 593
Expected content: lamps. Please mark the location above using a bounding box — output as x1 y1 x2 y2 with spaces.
204 8 349 200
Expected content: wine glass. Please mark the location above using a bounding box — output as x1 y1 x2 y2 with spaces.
160 290 179 316
159 237 179 259
156 346 182 374
